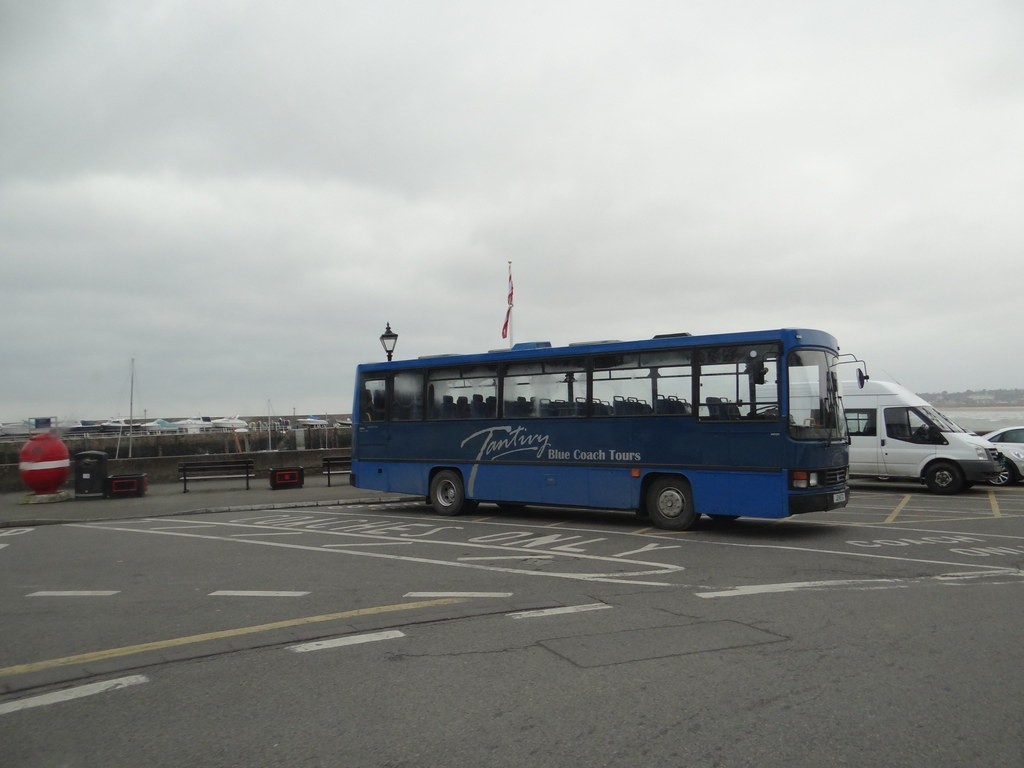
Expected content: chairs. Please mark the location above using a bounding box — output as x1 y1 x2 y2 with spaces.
539 399 573 416
365 389 413 420
657 394 692 415
614 395 650 417
576 397 610 416
438 394 498 419
705 397 732 419
511 396 536 417
720 398 742 419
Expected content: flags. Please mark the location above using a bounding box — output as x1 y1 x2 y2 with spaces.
502 271 513 339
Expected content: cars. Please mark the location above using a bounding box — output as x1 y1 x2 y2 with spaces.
978 427 1024 485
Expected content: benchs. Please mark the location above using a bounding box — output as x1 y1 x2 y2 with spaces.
177 460 256 494
322 457 352 487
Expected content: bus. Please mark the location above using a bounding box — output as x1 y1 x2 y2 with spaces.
349 326 870 532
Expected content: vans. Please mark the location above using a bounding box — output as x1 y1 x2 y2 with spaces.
752 380 1006 496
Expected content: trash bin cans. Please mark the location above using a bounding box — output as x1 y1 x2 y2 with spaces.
75 451 108 499
